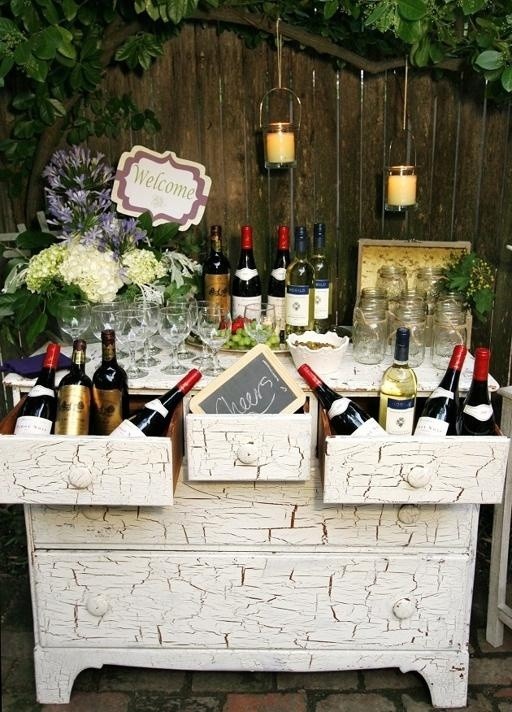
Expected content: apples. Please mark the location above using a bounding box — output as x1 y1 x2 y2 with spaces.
232 317 243 334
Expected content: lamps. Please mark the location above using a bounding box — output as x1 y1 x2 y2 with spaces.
258 87 302 169
382 129 421 212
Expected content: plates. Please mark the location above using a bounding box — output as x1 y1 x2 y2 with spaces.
185 324 354 355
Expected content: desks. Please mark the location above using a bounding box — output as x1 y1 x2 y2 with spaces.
0 325 508 708
483 385 512 647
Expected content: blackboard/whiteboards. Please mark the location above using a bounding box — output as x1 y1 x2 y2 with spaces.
188 343 307 415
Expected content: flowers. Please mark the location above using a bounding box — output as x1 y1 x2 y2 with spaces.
436 251 498 322
0 140 206 351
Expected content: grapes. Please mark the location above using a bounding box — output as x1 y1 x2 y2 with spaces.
218 323 278 350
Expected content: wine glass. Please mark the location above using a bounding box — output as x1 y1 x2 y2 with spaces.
57 294 276 380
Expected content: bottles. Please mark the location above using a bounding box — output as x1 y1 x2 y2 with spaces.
108 369 202 437
12 344 61 435
459 348 495 437
204 223 338 341
52 339 92 435
354 265 468 370
90 329 129 435
298 363 391 438
413 344 468 436
378 327 419 436
